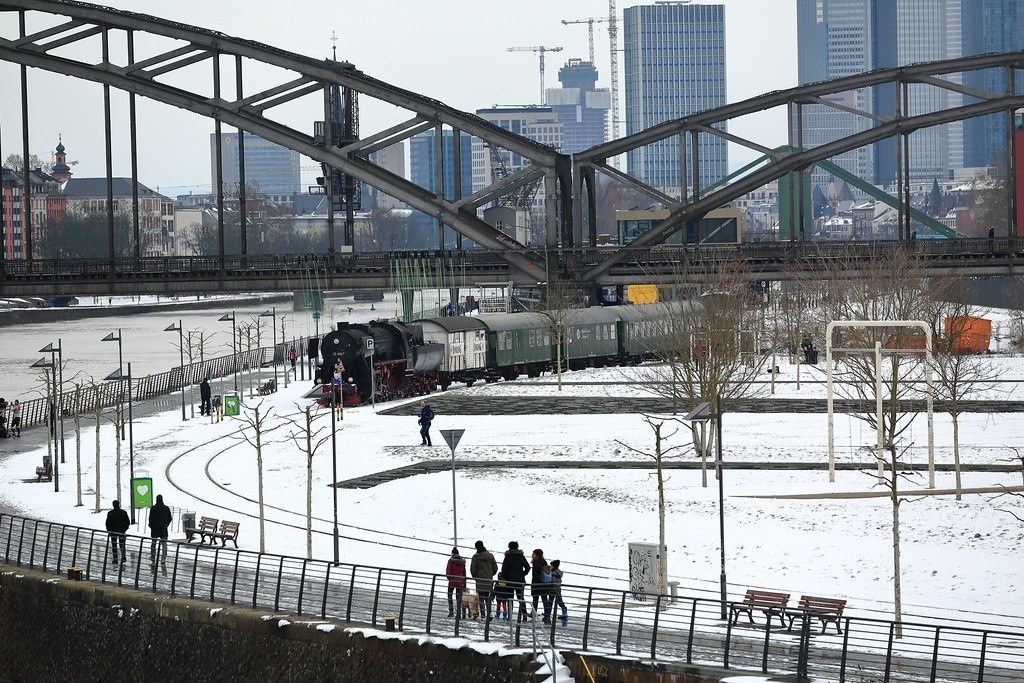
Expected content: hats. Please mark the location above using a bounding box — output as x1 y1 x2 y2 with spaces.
533 549 543 556
550 560 560 568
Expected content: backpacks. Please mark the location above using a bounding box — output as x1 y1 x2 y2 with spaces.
540 566 551 582
429 409 434 419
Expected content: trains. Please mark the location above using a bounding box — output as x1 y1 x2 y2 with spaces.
303 285 737 404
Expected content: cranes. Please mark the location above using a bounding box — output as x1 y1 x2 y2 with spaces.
506 46 564 107
18 150 80 174
561 16 623 67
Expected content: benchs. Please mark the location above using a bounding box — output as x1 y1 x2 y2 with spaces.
353 291 384 300
36 459 53 482
197 397 220 416
185 516 219 545
785 596 847 635
731 590 790 630
200 520 240 548
255 381 273 396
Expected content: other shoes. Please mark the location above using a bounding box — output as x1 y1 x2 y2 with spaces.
148 557 155 562
428 442 431 446
481 617 485 621
112 560 118 564
422 440 426 445
17 434 21 438
123 557 126 562
161 561 165 564
12 433 16 436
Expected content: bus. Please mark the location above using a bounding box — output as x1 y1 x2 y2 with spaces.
615 209 742 252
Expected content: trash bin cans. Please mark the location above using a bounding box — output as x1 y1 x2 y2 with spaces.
133 469 154 508
182 514 195 534
224 390 240 415
804 351 818 364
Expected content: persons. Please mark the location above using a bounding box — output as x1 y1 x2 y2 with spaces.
200 378 212 416
443 302 452 317
149 495 172 564
418 401 434 446
0 400 21 438
502 541 530 622
490 572 507 620
988 225 994 249
106 500 130 564
446 547 466 617
289 346 295 371
528 549 550 622
470 541 498 621
549 560 568 618
911 231 916 239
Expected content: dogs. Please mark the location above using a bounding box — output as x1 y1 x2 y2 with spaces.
461 588 480 620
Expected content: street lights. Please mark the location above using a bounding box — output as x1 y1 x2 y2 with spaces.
441 299 446 312
260 314 277 392
218 318 237 397
305 393 339 568
434 302 439 318
31 364 59 492
103 368 135 524
460 295 465 312
101 338 125 441
532 247 549 309
690 413 726 620
40 349 64 463
164 328 185 421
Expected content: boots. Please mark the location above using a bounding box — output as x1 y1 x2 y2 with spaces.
448 608 454 617
558 609 568 618
503 613 507 620
455 610 461 619
495 612 500 618
